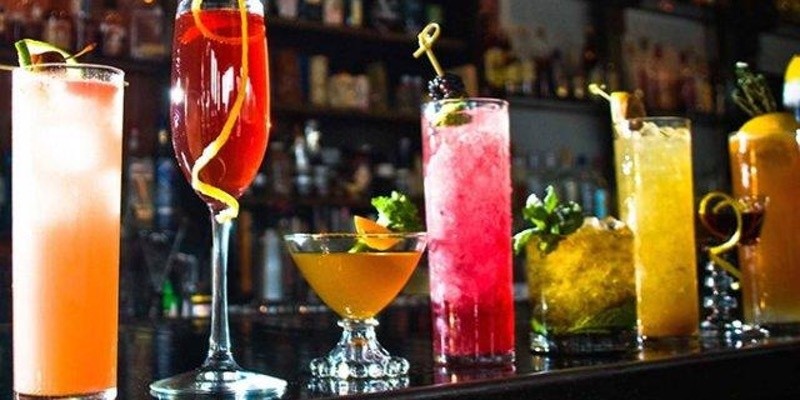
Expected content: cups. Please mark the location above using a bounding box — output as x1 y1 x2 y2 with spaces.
522 225 646 357
418 97 518 368
611 116 701 346
9 61 126 400
729 130 800 330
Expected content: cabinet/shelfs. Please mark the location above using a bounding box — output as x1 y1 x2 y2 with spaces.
233 14 474 301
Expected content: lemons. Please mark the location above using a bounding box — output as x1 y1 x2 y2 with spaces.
730 112 798 151
354 215 401 250
15 39 84 80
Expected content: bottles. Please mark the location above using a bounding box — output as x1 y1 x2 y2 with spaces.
1 0 726 322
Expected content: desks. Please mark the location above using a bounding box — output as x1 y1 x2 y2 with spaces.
0 297 799 400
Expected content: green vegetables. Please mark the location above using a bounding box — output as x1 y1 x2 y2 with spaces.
729 63 779 113
512 185 584 258
436 95 471 126
349 191 421 255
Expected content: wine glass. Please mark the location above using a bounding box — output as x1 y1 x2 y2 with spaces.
150 2 286 396
282 231 429 380
